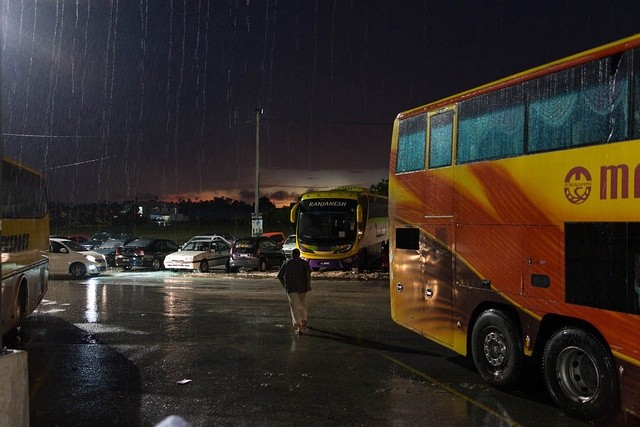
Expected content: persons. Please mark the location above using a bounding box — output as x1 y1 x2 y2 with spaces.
277 249 314 335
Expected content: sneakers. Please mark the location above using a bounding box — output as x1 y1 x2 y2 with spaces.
301 320 308 328
295 324 302 334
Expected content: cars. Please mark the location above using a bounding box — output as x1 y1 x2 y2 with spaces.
164 235 230 275
115 239 179 271
64 234 87 244
78 232 106 250
260 231 285 240
210 232 236 243
281 234 297 254
229 236 285 273
93 235 132 265
49 237 105 278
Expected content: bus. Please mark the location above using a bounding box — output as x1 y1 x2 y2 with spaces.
0 156 50 336
388 38 640 417
290 184 389 272
